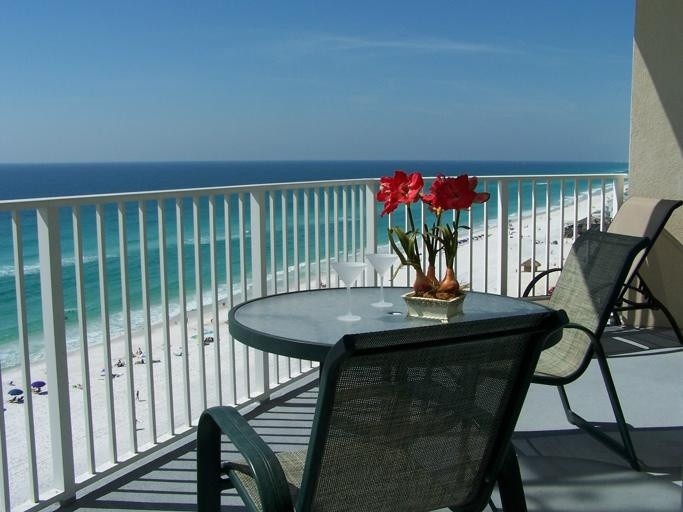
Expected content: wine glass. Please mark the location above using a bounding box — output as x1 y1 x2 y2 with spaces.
364 251 399 309
330 261 368 323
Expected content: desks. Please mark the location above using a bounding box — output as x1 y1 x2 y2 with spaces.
228 285 567 393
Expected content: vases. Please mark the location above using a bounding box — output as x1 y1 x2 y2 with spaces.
402 291 465 322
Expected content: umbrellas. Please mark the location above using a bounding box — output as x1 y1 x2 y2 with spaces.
31 381 46 388
9 389 23 395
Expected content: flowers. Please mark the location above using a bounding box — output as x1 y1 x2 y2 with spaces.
375 170 491 295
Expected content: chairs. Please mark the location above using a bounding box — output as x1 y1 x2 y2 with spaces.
606 198 683 347
195 310 571 511
519 229 651 472
